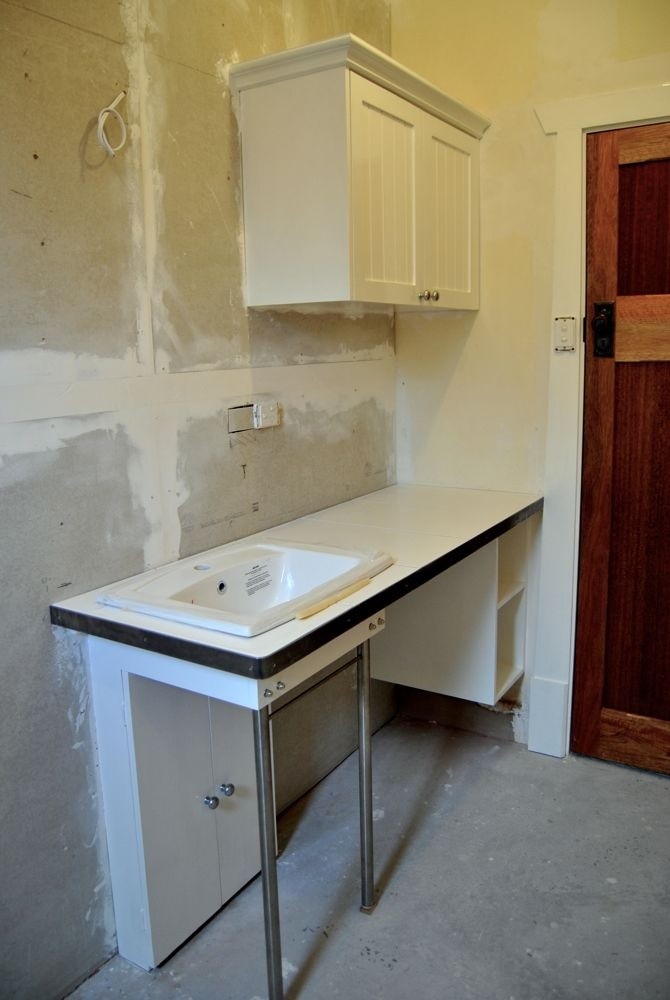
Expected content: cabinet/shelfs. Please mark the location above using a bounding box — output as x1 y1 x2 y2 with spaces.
87 633 278 972
351 516 534 710
228 32 494 315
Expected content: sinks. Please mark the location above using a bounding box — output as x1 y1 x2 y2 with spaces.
173 554 361 618
